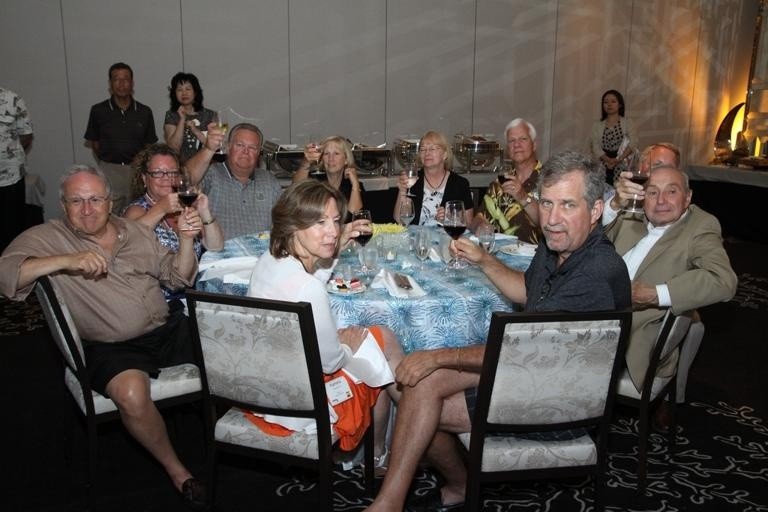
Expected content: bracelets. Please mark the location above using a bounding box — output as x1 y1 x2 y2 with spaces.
456 347 464 372
203 218 216 226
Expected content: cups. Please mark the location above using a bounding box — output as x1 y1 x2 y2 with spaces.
712 138 731 169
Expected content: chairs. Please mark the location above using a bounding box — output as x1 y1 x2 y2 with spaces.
184 288 375 510
32 276 204 510
457 307 632 512
469 189 479 210
617 306 692 493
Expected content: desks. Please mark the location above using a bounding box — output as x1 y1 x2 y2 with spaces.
277 170 513 222
25 173 46 231
687 164 768 204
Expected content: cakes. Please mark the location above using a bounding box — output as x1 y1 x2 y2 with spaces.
257 231 269 239
326 276 362 292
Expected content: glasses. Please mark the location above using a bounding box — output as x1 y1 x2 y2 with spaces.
144 170 180 178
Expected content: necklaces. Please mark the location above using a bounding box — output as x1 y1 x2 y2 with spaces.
424 169 447 189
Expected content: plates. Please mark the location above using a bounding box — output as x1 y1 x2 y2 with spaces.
499 243 537 259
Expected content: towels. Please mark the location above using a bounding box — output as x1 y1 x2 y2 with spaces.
337 324 395 387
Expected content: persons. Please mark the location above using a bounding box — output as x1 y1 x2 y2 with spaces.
118 143 224 300
246 180 406 471
84 64 158 216
393 131 473 232
590 89 638 182
0 87 34 256
293 135 366 223
163 72 219 164
600 166 739 394
638 143 680 176
474 117 545 245
0 163 208 508
359 151 632 512
183 123 284 245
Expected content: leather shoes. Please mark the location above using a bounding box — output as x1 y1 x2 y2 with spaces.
408 489 465 511
180 478 210 506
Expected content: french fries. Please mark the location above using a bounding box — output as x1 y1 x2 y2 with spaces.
370 223 406 235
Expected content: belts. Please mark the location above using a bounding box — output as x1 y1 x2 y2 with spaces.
101 159 133 165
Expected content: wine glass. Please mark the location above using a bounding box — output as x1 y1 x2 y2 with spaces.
498 166 513 205
625 147 648 215
325 200 494 299
400 161 421 201
208 106 228 159
175 172 201 232
307 135 325 178
